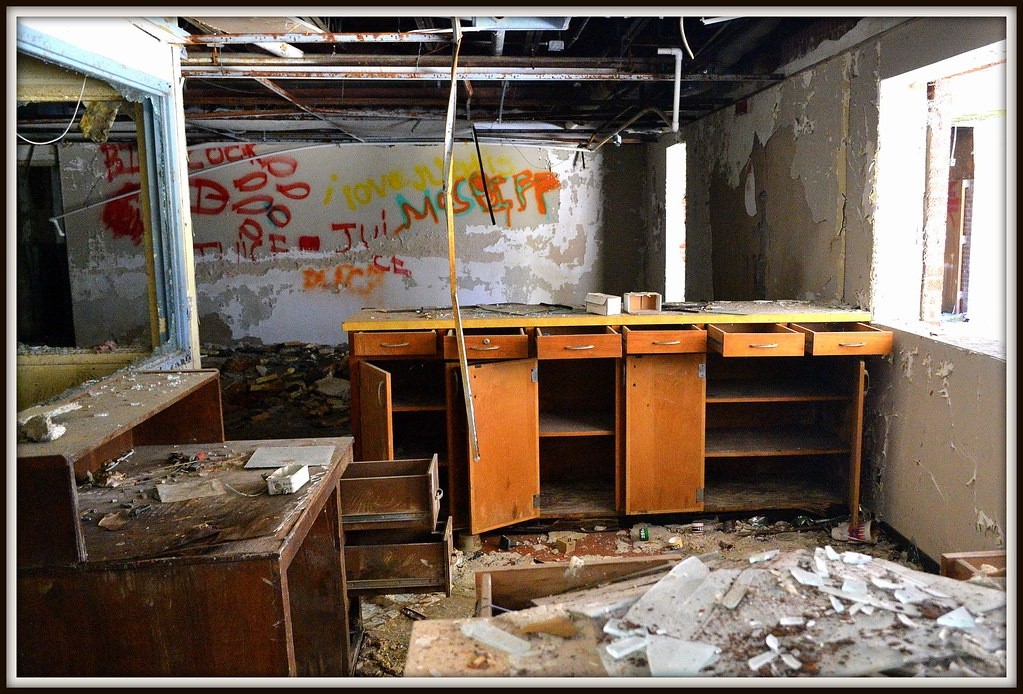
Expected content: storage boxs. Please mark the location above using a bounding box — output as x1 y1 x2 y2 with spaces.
584 293 622 315
623 292 663 314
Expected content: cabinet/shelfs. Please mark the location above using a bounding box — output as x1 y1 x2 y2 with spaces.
17 368 456 677
343 303 894 539
